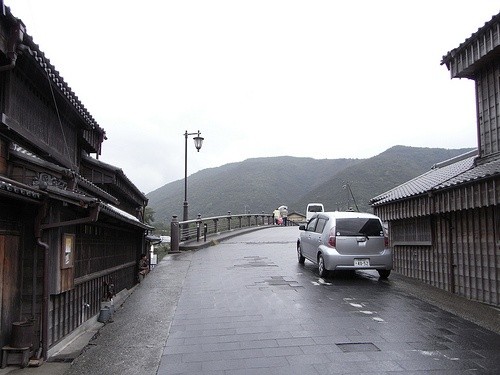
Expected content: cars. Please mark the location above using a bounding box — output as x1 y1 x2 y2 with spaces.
297 212 392 278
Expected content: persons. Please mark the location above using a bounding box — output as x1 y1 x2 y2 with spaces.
138 254 149 282
273 208 288 226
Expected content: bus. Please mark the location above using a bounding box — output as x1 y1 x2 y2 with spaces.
306 203 324 223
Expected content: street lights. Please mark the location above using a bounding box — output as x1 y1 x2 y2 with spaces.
181 130 204 242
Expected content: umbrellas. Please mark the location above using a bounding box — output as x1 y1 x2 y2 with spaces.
278 205 287 211
